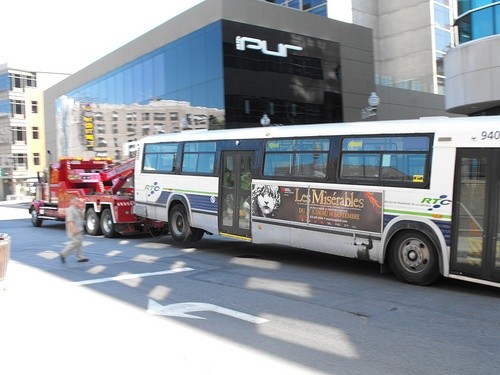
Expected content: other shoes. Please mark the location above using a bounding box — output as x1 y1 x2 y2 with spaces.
77 259 89 262
60 253 66 263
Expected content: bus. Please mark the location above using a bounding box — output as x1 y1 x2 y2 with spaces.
133 115 500 289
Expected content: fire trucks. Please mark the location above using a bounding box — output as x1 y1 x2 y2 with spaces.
30 153 169 239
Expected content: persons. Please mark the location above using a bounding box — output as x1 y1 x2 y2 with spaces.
312 161 326 178
60 197 89 263
